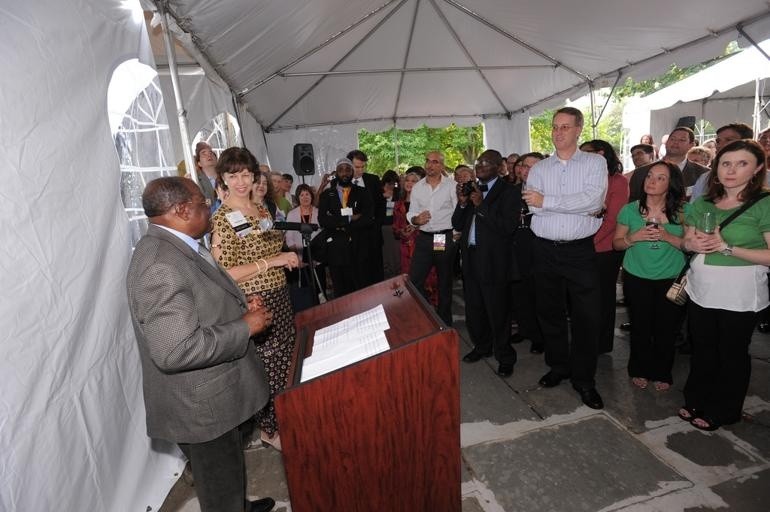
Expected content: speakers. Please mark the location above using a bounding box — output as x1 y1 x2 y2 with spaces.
292 142 316 177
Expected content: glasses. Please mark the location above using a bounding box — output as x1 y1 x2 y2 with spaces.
473 159 498 168
550 124 578 132
183 197 213 207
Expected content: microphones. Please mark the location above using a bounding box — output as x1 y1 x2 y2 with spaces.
260 219 319 233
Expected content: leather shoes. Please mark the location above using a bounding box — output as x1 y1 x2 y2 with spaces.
462 345 494 364
529 342 546 355
620 321 630 330
571 382 605 410
509 332 525 344
497 350 517 377
538 373 570 389
248 496 277 512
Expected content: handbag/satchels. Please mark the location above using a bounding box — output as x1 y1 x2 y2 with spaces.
665 274 691 307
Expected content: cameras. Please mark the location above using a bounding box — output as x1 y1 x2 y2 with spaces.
462 180 488 195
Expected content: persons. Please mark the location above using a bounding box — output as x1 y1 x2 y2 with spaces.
184 108 770 452
126 176 276 512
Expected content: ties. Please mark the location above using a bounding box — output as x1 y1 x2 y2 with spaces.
196 242 222 274
341 187 351 209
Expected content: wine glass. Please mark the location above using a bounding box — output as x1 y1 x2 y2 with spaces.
521 185 535 215
647 217 661 249
702 212 715 233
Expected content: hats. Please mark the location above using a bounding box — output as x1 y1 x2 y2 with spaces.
336 158 354 168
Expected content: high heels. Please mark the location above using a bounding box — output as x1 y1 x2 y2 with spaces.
260 430 284 453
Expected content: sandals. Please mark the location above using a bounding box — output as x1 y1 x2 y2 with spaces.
690 406 738 431
653 380 671 393
631 377 650 390
678 400 706 422
